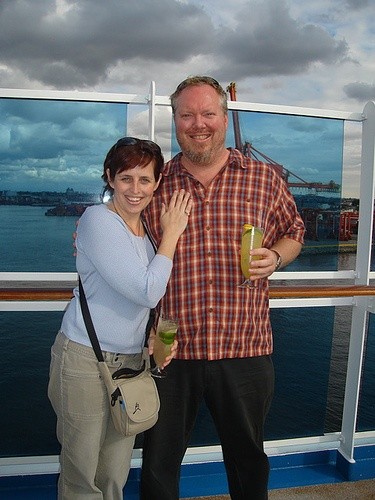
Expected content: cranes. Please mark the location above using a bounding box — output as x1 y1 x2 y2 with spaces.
225 82 344 192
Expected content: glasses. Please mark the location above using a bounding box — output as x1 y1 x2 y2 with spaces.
112 137 161 166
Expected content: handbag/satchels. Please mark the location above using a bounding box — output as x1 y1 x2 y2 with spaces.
109 367 160 437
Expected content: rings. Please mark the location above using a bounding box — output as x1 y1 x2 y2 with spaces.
184 209 190 217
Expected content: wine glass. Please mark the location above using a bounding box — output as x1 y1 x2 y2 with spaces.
146 317 180 379
238 226 265 289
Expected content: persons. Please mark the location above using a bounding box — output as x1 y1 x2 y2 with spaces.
47 136 193 500
72 76 305 500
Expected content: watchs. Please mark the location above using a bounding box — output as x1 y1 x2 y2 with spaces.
270 249 282 272
